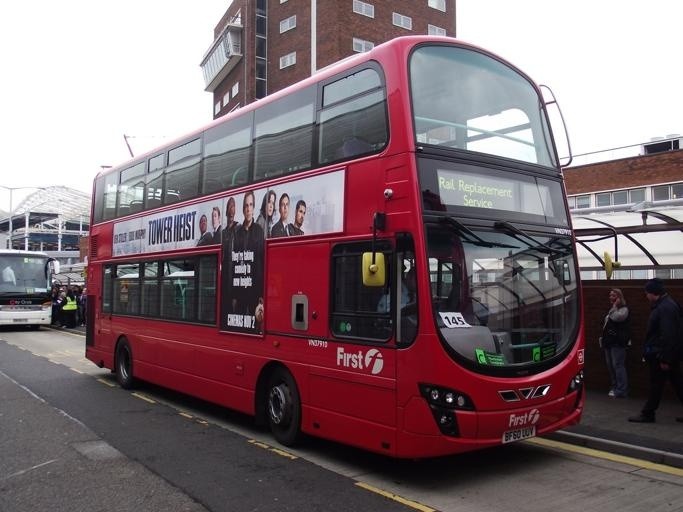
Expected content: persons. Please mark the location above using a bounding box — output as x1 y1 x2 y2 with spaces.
1 262 16 287
626 277 682 424
221 196 241 248
210 206 222 244
195 214 212 246
221 189 264 335
270 192 291 238
595 287 632 400
49 281 87 329
376 267 416 314
256 190 277 237
284 200 306 236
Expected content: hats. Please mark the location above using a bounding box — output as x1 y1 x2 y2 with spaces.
645 278 663 292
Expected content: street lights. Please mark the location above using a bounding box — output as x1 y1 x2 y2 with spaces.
7 186 87 249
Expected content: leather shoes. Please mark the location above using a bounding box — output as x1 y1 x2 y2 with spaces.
676 416 683 422
628 414 656 423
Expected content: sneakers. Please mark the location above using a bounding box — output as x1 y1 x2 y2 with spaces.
608 389 614 397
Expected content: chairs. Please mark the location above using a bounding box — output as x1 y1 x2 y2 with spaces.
98 119 381 218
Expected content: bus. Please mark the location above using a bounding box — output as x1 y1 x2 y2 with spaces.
0 247 60 329
80 33 590 462
